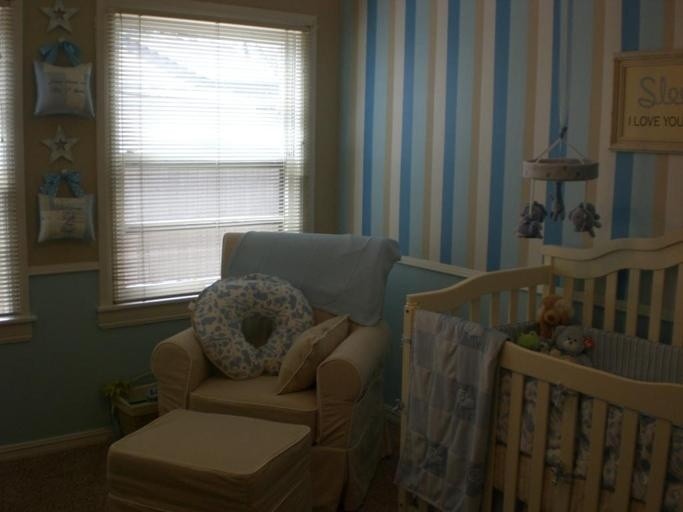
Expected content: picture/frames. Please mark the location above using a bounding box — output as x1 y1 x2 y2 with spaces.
607 48 683 155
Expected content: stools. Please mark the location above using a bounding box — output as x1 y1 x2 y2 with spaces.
106 407 315 510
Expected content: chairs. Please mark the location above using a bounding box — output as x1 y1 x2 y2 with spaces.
147 232 395 511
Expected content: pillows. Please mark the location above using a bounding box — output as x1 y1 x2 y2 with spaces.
193 273 351 396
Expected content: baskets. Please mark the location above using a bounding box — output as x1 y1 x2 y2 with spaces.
108 383 159 436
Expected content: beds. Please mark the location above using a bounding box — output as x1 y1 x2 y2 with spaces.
403 230 681 511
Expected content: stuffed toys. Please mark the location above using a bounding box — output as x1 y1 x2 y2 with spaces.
540 296 574 331
518 332 542 350
514 124 601 239
557 323 592 365
